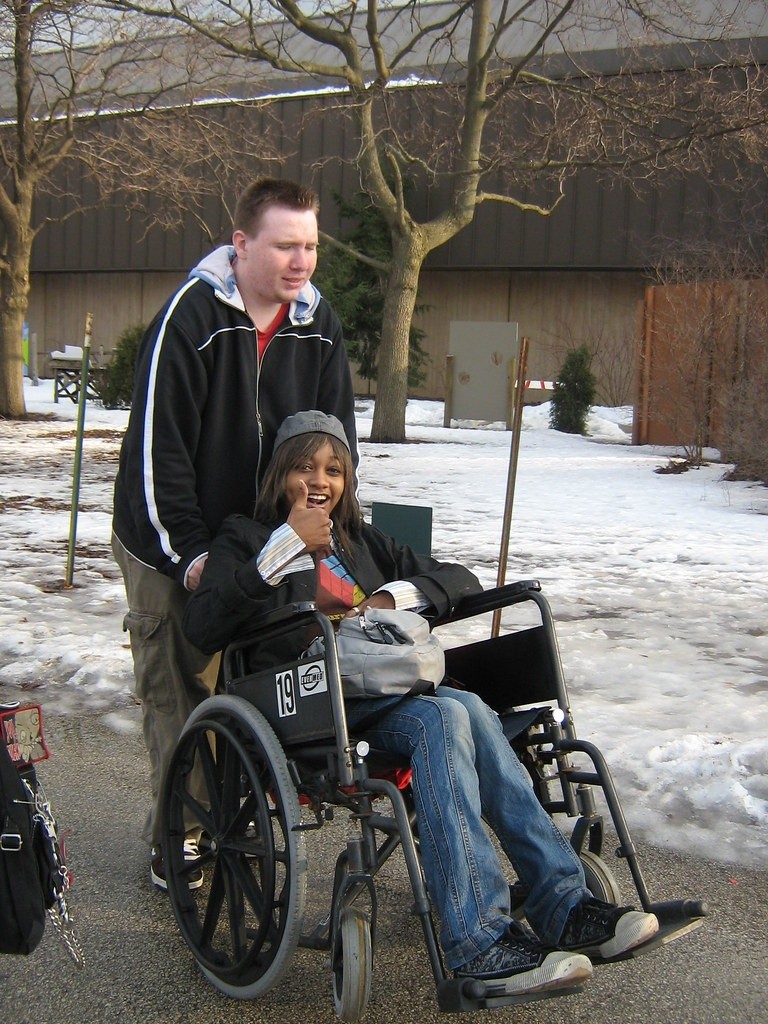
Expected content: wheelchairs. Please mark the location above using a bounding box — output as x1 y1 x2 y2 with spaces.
155 601 713 1023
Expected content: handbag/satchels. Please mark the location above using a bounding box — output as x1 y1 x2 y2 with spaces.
315 605 445 748
0 698 86 970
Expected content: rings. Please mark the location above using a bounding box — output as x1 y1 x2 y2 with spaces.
352 607 360 613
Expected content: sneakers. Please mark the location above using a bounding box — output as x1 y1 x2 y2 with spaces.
454 920 593 999
556 892 659 960
150 838 203 891
244 821 258 858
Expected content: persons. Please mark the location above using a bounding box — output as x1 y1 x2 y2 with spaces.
112 176 358 890
181 410 659 998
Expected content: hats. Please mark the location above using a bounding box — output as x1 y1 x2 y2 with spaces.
272 410 351 456
